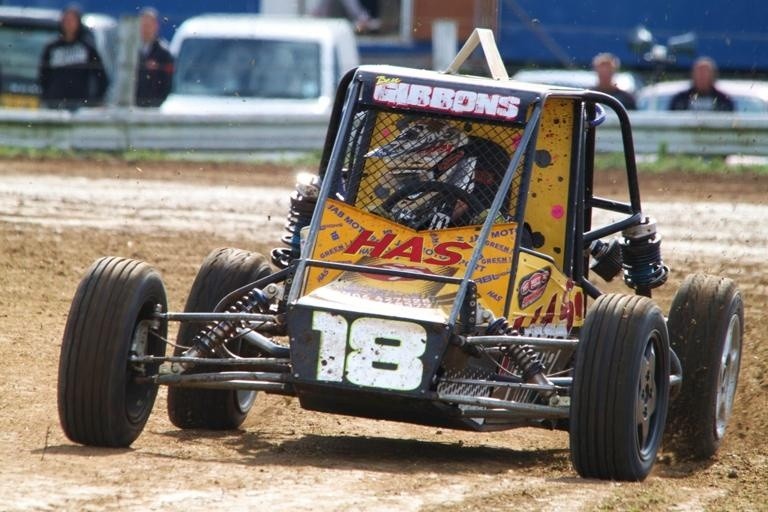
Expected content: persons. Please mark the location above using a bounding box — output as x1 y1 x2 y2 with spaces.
668 56 735 111
362 120 531 248
135 8 175 111
584 52 636 110
34 5 111 106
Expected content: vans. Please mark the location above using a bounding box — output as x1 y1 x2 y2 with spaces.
156 11 359 120
0 8 120 113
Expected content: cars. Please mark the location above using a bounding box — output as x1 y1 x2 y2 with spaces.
636 75 767 113
512 65 636 108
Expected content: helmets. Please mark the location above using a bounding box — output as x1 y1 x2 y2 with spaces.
363 124 477 221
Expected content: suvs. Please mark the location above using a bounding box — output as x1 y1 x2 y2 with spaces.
56 28 745 485
